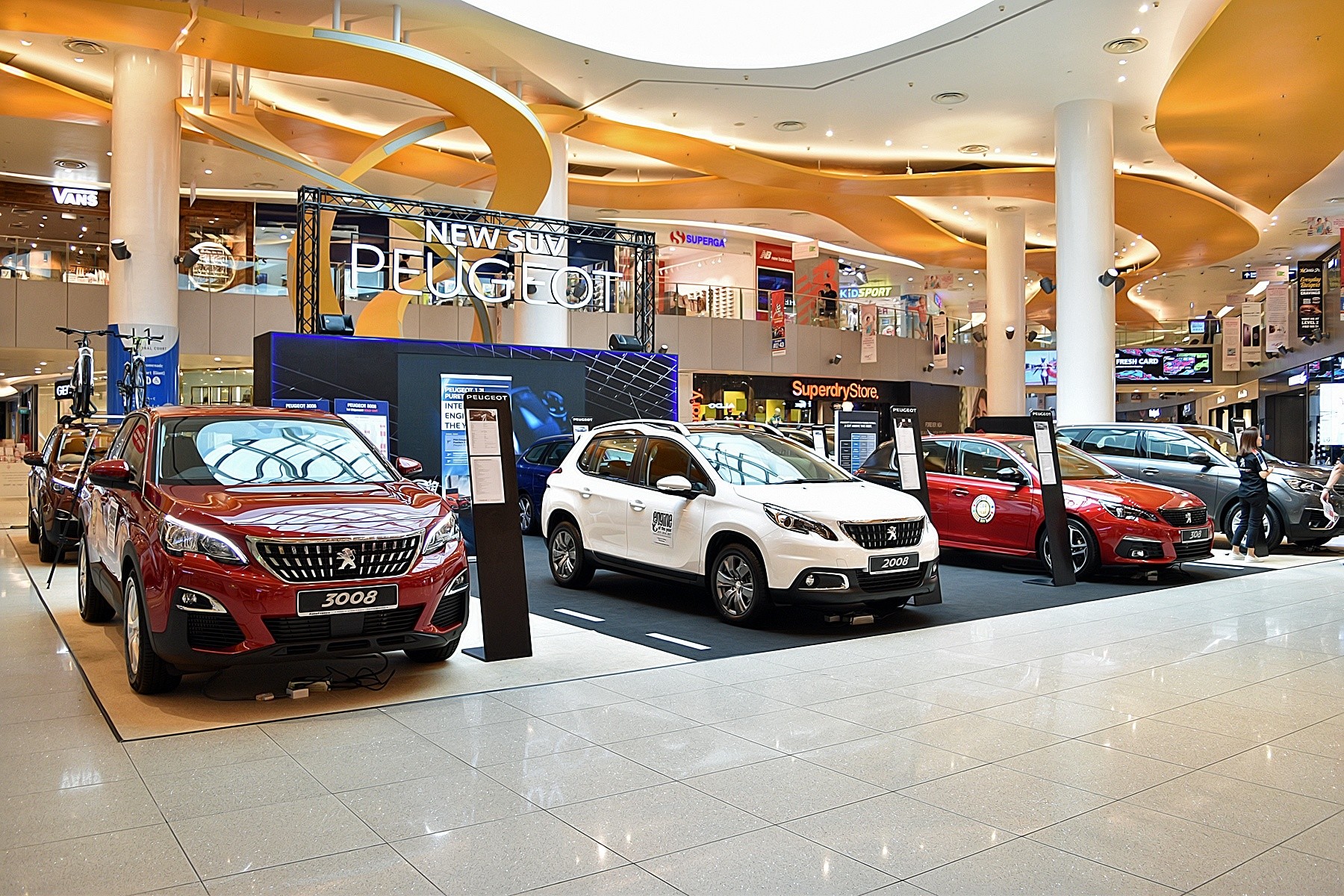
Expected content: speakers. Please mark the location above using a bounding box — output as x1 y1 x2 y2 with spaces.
609 334 643 350
316 314 354 336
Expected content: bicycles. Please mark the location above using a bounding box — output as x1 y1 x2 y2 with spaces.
56 326 116 417
113 328 164 415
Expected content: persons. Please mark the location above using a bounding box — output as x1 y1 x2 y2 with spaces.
1034 358 1053 385
1202 310 1217 344
1320 453 1344 504
864 316 872 333
909 276 935 338
1231 426 1274 562
399 255 410 283
970 389 987 430
815 283 837 329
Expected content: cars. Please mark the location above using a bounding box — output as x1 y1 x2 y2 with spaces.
753 427 834 457
445 493 471 510
855 433 1215 580
515 433 701 536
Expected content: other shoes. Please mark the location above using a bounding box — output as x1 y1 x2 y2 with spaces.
1244 553 1264 563
1230 552 1245 560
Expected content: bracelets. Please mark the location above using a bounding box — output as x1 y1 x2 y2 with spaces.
1323 487 1332 489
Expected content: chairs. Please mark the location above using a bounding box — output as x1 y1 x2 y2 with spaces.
162 435 206 478
965 448 995 477
650 444 688 484
62 438 86 454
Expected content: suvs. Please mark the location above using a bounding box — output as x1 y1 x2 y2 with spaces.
541 418 940 625
23 415 148 563
78 405 471 695
1056 423 1344 552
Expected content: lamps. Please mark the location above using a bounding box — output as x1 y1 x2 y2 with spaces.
1026 330 1038 342
1277 344 1294 355
829 354 842 365
1005 326 1014 339
1300 329 1329 346
972 331 987 343
1115 277 1125 294
923 363 934 372
1040 277 1056 294
566 282 586 298
1247 362 1260 367
1265 352 1279 359
953 366 965 375
173 248 201 268
1159 394 1168 400
527 276 537 294
658 344 668 354
111 239 132 260
1099 268 1118 286
1177 392 1185 396
